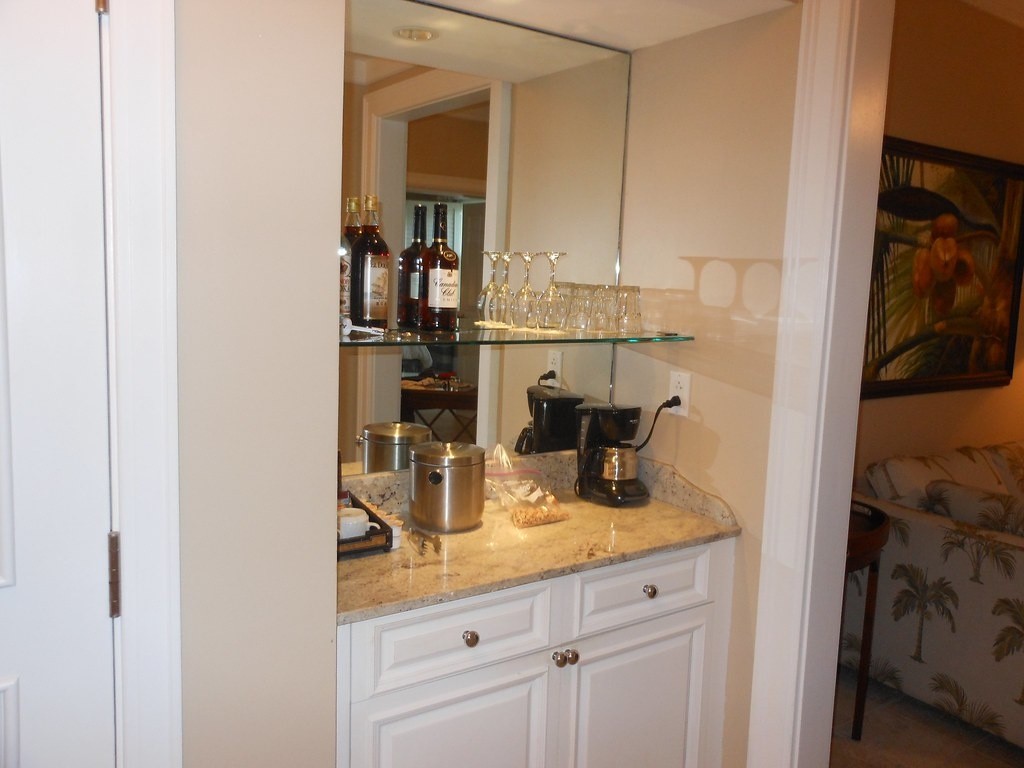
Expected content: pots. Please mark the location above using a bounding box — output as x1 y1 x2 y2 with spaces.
355 420 431 473
409 441 486 531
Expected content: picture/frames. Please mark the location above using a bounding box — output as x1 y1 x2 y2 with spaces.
860 134 1024 400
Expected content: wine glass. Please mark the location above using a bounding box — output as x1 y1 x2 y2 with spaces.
474 251 503 325
510 252 542 329
539 251 566 329
489 252 518 328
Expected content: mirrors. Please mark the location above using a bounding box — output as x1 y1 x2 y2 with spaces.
338 0 631 476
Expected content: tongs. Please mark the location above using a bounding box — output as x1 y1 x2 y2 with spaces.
407 527 442 554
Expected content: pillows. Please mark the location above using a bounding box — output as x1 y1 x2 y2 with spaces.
865 446 1010 517
926 479 1024 537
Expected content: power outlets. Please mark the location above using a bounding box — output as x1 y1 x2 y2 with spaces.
545 348 564 387
667 370 690 416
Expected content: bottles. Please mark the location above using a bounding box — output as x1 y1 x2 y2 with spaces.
343 197 364 246
396 204 432 332
417 202 461 335
340 231 353 318
350 193 390 330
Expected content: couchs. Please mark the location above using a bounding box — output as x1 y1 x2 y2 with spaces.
837 439 1024 748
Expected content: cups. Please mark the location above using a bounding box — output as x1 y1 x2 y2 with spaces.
617 286 642 334
361 498 404 549
551 282 576 316
587 285 621 333
338 507 381 538
567 284 598 331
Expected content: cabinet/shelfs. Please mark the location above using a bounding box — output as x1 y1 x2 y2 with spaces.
337 535 737 767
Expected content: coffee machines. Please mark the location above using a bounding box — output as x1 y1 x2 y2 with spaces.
511 385 585 455
575 402 651 506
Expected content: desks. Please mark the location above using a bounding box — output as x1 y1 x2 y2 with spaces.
400 377 478 444
